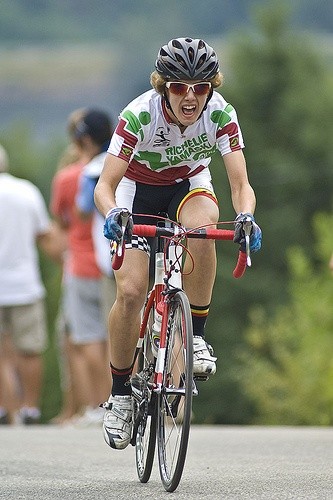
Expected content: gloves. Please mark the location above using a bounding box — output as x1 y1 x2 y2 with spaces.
103 207 133 245
232 212 262 254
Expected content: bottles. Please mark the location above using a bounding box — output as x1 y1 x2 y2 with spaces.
152 301 164 359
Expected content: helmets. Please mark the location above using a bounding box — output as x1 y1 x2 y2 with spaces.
154 37 221 83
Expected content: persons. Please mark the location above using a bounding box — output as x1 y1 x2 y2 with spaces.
93 37 263 449
0 144 64 429
51 108 137 430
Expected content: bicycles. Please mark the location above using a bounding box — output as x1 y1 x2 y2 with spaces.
111 209 253 492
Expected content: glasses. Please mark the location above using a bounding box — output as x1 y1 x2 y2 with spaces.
164 80 210 97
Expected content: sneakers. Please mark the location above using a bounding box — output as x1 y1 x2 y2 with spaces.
183 337 218 376
99 392 135 450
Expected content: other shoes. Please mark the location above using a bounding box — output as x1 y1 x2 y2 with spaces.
0 408 46 424
76 406 105 427
